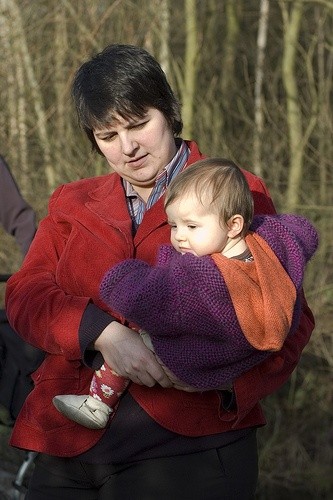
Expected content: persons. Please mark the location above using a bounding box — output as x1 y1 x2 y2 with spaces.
5 44 315 500
52 158 318 430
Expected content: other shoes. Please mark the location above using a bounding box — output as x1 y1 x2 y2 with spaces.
52 394 113 430
